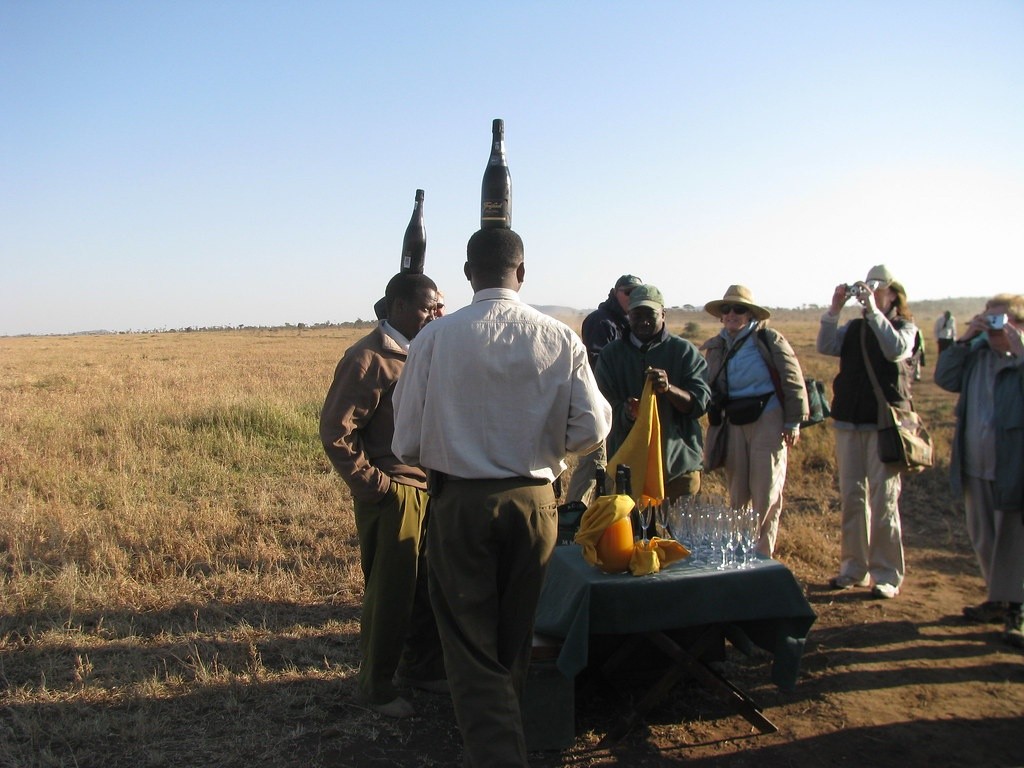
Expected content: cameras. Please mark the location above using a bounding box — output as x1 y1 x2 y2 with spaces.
845 286 859 296
985 314 1009 330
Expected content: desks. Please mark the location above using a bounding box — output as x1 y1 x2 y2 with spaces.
533 542 817 749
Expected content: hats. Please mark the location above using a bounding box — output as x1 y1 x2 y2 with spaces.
705 285 771 320
628 284 665 311
866 265 907 297
615 275 642 291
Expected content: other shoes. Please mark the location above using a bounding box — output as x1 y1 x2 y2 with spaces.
372 696 413 720
916 374 921 379
873 582 899 599
1003 608 1024 631
829 572 871 588
963 601 1006 623
410 676 449 698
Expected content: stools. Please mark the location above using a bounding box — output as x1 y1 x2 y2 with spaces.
522 635 575 751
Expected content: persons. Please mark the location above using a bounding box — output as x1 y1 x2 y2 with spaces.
910 330 924 381
565 274 643 505
320 271 445 718
594 286 712 502
934 293 1024 628
392 228 613 768
817 264 922 598
699 284 810 559
935 310 956 353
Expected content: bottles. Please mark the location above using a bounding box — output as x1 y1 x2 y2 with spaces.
595 464 631 499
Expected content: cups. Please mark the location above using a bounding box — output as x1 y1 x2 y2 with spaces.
595 512 633 574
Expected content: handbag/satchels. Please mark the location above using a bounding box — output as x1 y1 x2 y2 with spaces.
799 379 831 429
877 405 932 474
704 417 728 472
723 391 776 426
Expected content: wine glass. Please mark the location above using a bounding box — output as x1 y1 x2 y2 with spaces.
637 498 652 542
655 497 670 539
667 494 763 571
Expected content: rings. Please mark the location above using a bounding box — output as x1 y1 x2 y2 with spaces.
1009 333 1013 336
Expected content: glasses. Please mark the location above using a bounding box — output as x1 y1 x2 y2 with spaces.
623 288 632 296
720 303 749 314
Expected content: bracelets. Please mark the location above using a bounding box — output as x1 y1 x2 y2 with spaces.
668 384 671 391
